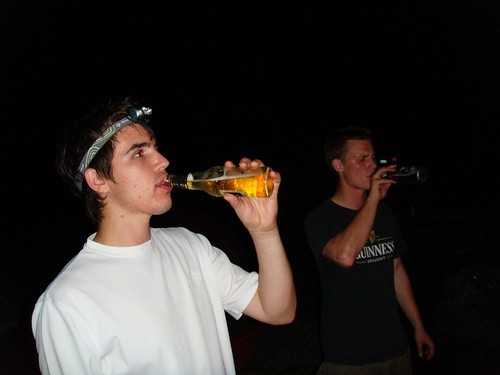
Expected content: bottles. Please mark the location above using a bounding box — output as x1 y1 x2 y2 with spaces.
162 166 275 198
376 166 420 181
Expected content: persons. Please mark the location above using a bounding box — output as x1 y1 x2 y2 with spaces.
31 100 297 375
305 127 435 375
